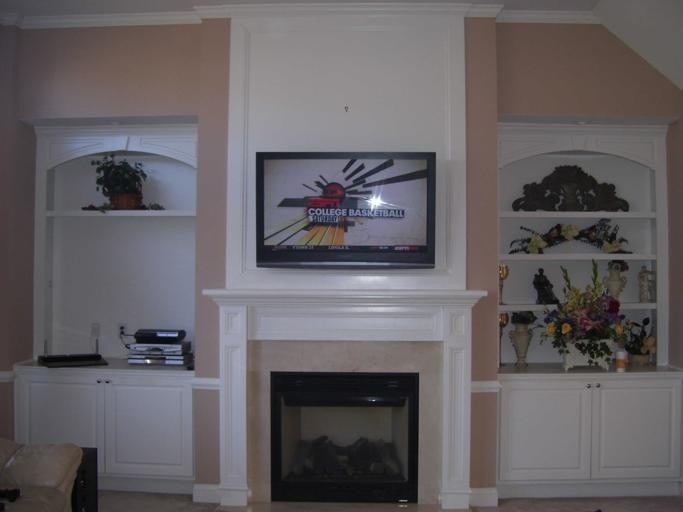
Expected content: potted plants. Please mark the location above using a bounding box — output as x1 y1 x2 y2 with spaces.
85 153 149 210
627 319 652 366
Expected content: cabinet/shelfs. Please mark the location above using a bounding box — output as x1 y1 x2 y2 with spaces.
492 119 676 371
29 122 201 375
14 363 193 496
493 373 683 500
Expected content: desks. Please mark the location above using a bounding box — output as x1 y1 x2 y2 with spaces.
70 445 95 512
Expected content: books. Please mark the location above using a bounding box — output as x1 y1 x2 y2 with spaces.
125 341 193 367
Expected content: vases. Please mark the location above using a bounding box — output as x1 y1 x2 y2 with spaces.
506 321 531 368
557 336 611 372
601 260 624 298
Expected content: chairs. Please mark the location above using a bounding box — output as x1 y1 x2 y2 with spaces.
0 438 100 511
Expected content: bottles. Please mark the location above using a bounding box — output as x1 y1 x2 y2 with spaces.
615 349 628 375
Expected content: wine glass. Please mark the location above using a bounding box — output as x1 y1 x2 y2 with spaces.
498 266 509 306
498 312 509 369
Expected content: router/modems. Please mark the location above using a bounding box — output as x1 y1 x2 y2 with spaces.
41 338 102 361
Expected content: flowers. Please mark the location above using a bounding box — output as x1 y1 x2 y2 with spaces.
505 218 632 256
522 253 631 368
